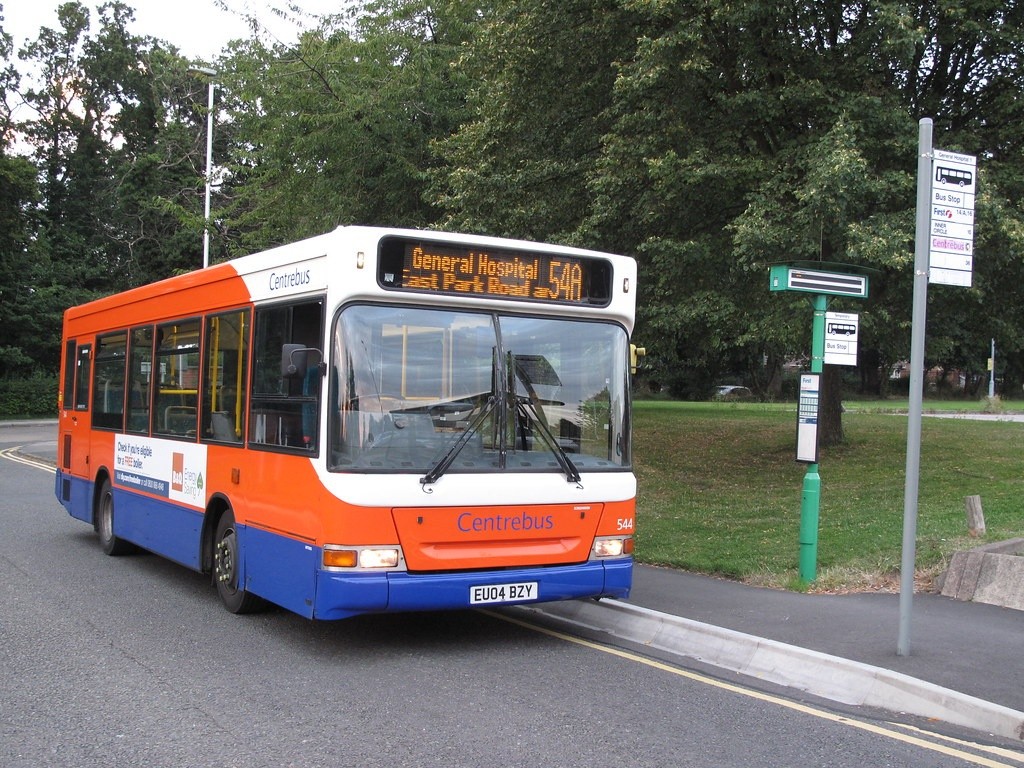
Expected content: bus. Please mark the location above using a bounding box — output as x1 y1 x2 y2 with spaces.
53 224 647 624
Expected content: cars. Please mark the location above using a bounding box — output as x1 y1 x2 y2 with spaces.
714 385 752 400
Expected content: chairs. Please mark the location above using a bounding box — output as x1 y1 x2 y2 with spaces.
103 378 244 442
300 367 372 448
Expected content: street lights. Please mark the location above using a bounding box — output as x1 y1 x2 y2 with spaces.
188 64 217 273
